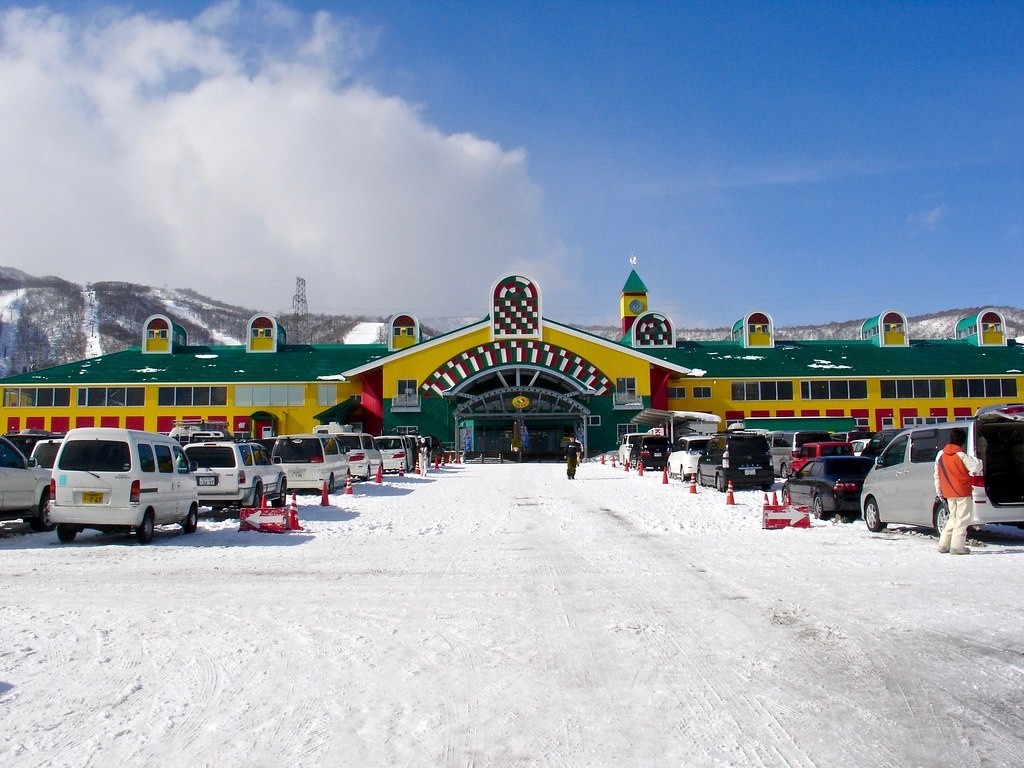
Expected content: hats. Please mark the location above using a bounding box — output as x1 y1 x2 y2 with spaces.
418 436 423 438
421 438 425 443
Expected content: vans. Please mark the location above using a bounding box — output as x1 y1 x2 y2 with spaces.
0 419 431 545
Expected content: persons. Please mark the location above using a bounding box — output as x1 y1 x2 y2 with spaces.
564 436 583 479
933 428 983 555
417 435 431 477
463 431 471 451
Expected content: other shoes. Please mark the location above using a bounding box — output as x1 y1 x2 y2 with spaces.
424 473 426 477
938 546 948 553
949 547 970 555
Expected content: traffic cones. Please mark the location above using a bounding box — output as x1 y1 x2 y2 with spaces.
785 492 792 505
449 453 453 463
601 453 644 476
688 471 698 492
772 491 779 505
319 480 331 506
286 492 302 530
414 459 420 474
764 493 770 505
398 462 405 476
661 465 670 483
726 479 736 504
260 494 267 509
441 454 445 465
345 473 353 494
434 457 439 469
375 465 382 483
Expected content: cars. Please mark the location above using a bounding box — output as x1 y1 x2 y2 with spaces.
618 400 1024 542
